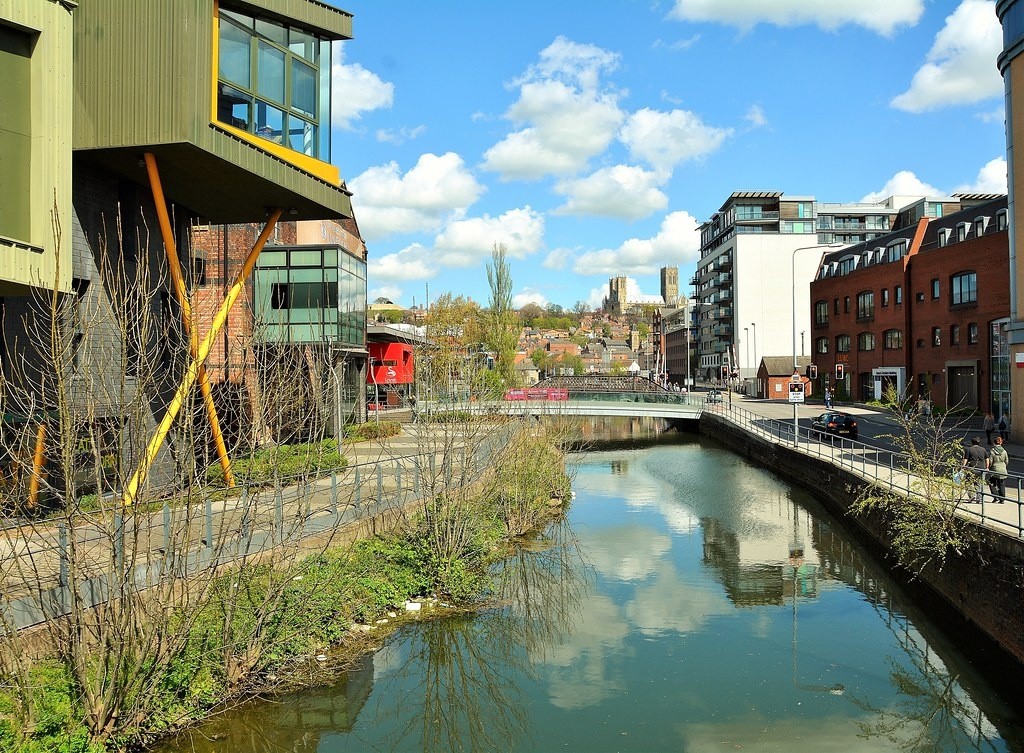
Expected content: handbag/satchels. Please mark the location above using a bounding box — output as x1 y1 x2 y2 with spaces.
999 416 1006 430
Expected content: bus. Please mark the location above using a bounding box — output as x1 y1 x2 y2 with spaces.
504 388 568 400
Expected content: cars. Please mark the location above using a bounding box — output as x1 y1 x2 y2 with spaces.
706 390 723 403
811 411 858 441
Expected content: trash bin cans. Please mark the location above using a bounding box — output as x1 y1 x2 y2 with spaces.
917 399 934 417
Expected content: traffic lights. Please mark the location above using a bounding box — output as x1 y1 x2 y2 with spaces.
835 364 844 380
810 366 817 379
721 366 728 381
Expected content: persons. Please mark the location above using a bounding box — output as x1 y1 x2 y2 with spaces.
998 412 1011 444
825 388 831 409
983 412 995 445
908 401 935 424
985 437 1009 504
960 437 990 504
411 394 416 407
668 382 686 403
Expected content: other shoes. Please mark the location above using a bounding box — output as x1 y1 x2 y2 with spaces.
968 496 975 503
998 500 1004 504
992 498 998 503
976 499 982 504
987 442 991 445
1003 441 1008 444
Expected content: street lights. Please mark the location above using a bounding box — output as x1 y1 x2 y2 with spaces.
792 242 844 447
790 482 846 696
687 301 712 405
647 332 661 388
